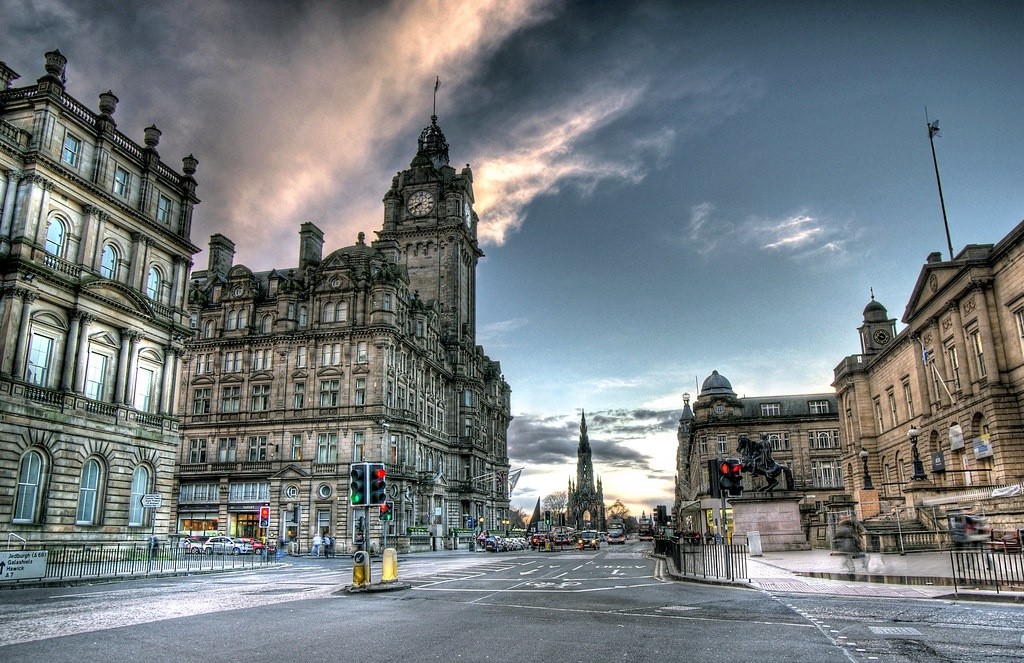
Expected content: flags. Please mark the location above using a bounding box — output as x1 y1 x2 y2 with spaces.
921 344 928 366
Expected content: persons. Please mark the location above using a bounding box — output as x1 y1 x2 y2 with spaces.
310 532 336 559
749 432 772 477
832 513 872 574
276 536 284 559
653 530 722 556
950 504 994 572
148 536 158 560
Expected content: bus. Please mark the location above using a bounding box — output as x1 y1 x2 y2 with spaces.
607 522 627 544
638 518 654 542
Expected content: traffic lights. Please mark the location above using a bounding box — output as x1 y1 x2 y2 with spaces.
350 464 368 507
379 501 394 521
545 511 550 525
721 458 744 498
259 507 270 529
368 463 387 507
714 457 734 499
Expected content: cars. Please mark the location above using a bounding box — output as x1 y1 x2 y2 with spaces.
184 538 203 554
482 535 533 553
202 536 248 555
238 538 275 555
552 530 607 551
531 534 550 548
